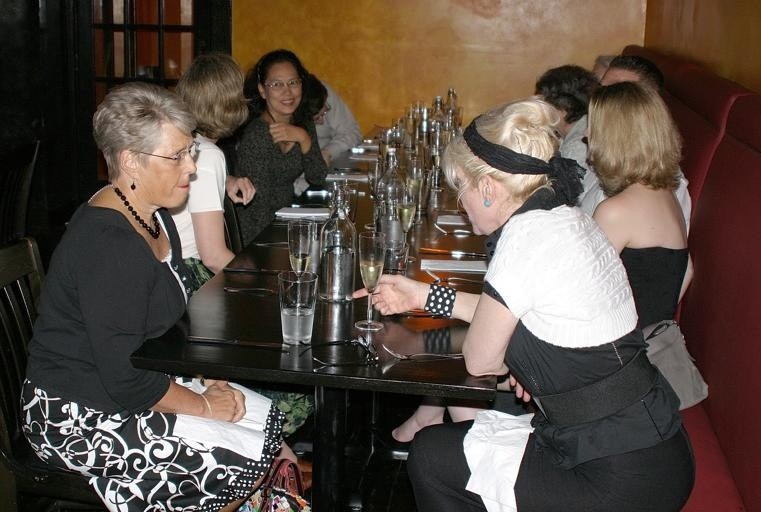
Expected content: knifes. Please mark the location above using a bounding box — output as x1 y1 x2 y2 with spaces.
222 266 287 274
419 246 488 254
186 336 283 350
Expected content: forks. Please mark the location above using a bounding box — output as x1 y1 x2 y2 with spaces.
434 223 473 236
381 344 464 361
424 268 486 285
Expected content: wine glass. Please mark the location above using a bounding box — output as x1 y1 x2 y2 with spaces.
286 219 317 315
396 203 417 265
367 100 463 204
326 181 359 226
354 231 387 333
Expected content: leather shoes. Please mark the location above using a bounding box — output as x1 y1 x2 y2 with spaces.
370 428 411 455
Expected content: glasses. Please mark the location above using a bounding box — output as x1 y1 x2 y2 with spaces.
299 335 380 369
128 141 198 163
264 80 303 89
312 104 333 123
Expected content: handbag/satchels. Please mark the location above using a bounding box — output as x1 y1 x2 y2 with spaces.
644 320 709 411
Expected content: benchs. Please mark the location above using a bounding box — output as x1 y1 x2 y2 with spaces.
623 44 761 512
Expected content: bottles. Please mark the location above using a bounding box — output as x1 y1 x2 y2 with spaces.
378 148 407 243
421 102 428 132
446 89 457 110
432 95 445 145
405 102 416 149
320 186 358 301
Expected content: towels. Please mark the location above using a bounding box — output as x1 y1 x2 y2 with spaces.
275 206 331 220
358 142 381 151
464 410 533 512
436 213 468 227
326 171 371 183
349 153 380 161
173 377 273 462
420 259 488 273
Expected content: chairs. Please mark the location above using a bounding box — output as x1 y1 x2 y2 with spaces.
1 234 108 511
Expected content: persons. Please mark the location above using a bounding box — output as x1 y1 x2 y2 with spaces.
350 95 698 512
232 47 330 250
288 74 365 197
596 51 670 95
17 82 313 511
531 63 609 219
372 79 694 454
168 50 258 275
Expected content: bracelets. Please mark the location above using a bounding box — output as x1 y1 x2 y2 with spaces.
199 393 212 415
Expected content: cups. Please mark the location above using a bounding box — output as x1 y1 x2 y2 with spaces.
276 269 320 346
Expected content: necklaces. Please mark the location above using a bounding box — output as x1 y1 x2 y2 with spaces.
266 108 278 125
112 184 163 239
